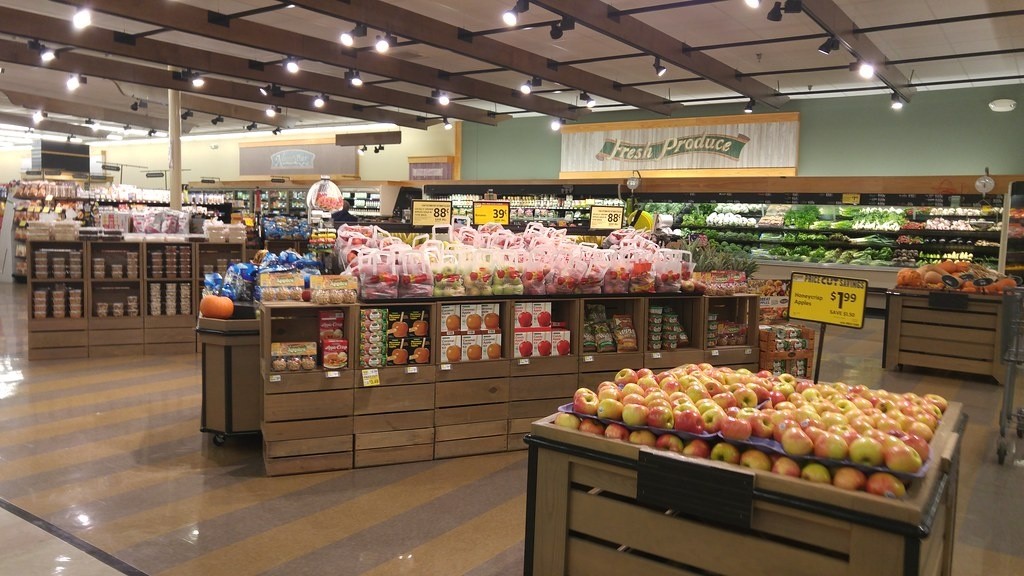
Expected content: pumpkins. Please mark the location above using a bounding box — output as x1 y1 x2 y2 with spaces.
200 292 234 319
896 260 1016 294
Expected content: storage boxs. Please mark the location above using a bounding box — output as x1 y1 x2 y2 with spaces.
269 309 349 372
206 224 247 242
758 324 815 379
359 309 430 369
310 275 358 290
264 240 298 254
260 272 305 287
515 302 571 358
297 240 308 255
440 304 502 363
15 212 129 277
407 155 453 180
691 270 792 322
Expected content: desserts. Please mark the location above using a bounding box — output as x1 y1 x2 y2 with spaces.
390 310 569 364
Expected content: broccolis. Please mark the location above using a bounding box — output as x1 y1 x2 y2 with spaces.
713 203 767 212
706 212 758 254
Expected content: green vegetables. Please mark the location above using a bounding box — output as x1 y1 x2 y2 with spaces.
768 216 908 265
689 203 717 215
787 205 904 217
644 203 686 214
681 215 706 240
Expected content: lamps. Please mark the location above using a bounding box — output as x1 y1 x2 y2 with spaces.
272 127 282 135
123 124 131 130
315 93 329 107
33 112 48 123
375 33 398 54
374 144 384 154
973 166 995 196
743 97 756 114
266 104 282 118
502 0 530 27
653 55 667 77
625 169 642 190
282 54 299 73
345 68 362 86
340 25 368 47
843 56 874 78
66 134 76 143
181 68 204 88
247 122 257 131
520 75 542 96
68 72 88 90
767 0 802 21
551 118 567 131
357 145 367 155
258 84 271 97
130 100 148 112
74 5 90 29
181 109 193 120
890 91 903 111
148 129 157 137
211 115 224 125
550 15 575 40
83 118 96 126
817 34 840 56
580 92 595 108
431 89 450 107
26 38 60 63
441 117 453 130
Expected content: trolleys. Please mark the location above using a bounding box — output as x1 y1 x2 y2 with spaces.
995 286 1023 467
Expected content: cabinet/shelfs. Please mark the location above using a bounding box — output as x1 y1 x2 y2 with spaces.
196 241 246 353
254 292 761 479
882 283 1006 387
87 240 145 359
519 400 968 576
142 242 195 356
25 238 88 360
194 310 261 446
0 197 30 283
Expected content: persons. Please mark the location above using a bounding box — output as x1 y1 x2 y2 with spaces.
331 199 356 226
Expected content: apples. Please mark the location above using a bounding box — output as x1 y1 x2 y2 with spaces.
302 290 311 301
338 225 706 296
553 363 947 498
